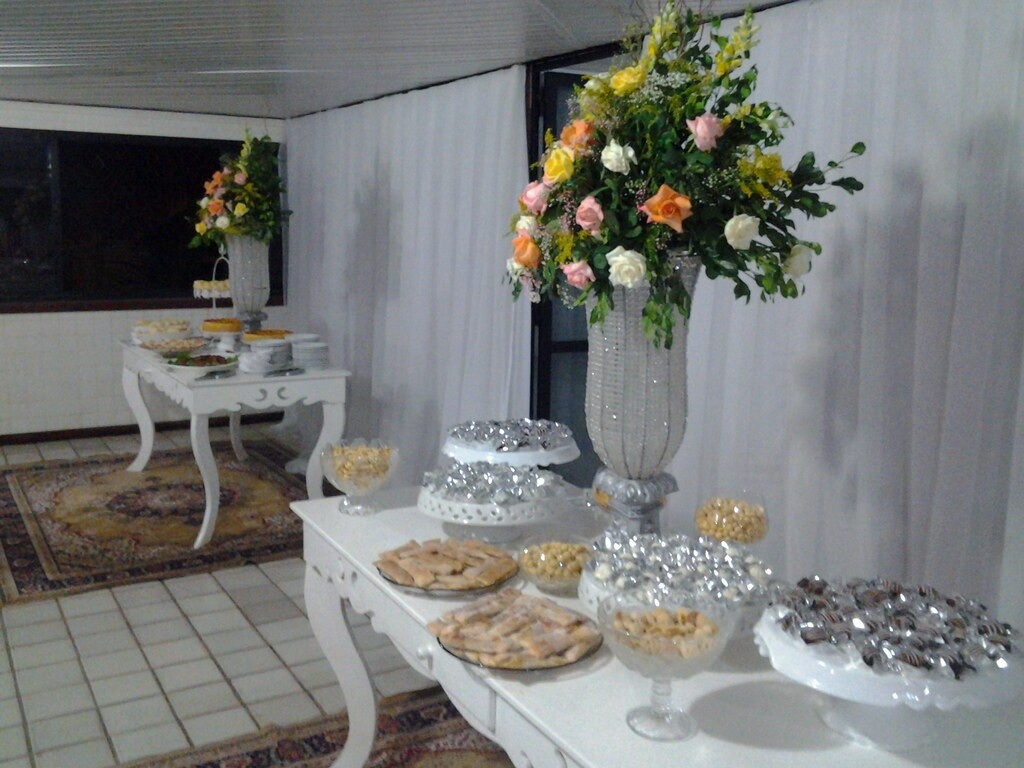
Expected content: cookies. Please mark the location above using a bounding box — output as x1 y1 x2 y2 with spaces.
425 587 602 668
372 537 519 590
331 444 393 492
132 317 210 348
612 610 722 663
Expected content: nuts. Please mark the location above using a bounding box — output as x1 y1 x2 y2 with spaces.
697 498 769 544
524 539 590 591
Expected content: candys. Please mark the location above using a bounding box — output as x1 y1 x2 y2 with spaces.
767 574 1016 684
423 416 573 506
590 526 771 607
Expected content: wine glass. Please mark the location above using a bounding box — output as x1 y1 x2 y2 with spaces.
597 586 734 742
320 438 400 515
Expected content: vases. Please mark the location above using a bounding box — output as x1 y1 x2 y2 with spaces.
579 254 700 618
227 236 270 355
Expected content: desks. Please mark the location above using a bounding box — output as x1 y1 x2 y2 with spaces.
120 339 352 549
289 486 1024 768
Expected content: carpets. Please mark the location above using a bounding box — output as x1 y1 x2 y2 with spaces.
101 681 515 768
0 440 345 609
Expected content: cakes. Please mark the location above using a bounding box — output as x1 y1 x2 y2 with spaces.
193 278 294 342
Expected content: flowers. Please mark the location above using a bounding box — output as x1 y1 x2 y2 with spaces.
498 1 864 349
189 123 295 256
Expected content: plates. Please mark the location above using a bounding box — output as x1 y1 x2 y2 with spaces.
250 333 328 372
201 330 242 341
161 353 239 373
376 566 519 597
437 605 604 673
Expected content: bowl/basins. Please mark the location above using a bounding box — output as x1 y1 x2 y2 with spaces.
134 335 210 356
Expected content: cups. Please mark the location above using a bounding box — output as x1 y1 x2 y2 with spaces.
689 487 771 557
